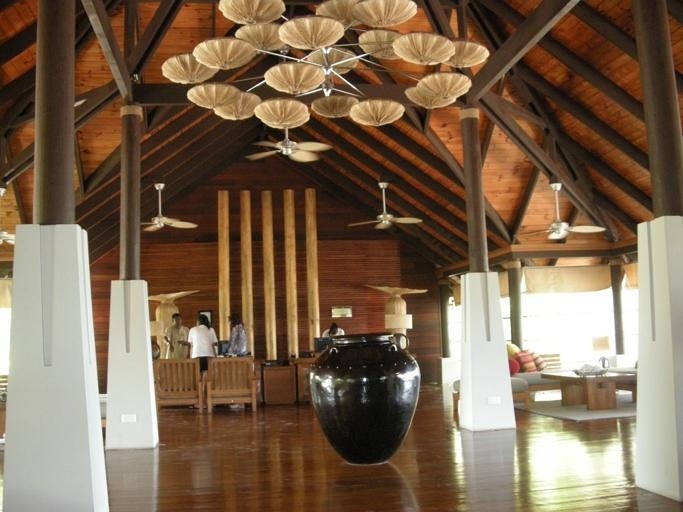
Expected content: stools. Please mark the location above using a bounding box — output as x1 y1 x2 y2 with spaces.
452 377 531 412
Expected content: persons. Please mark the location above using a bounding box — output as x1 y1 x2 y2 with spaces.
321 322 344 337
227 312 247 356
187 313 219 371
163 313 190 360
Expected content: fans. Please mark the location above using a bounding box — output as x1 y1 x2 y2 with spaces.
515 181 606 239
346 182 423 230
244 127 332 164
140 182 199 232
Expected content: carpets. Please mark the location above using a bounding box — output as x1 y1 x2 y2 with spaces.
513 392 636 423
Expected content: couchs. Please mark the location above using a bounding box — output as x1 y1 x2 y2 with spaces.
508 354 581 393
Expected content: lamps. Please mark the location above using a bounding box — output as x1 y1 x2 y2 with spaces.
159 0 490 130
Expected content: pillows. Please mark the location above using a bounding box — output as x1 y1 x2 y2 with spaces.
508 359 520 374
528 352 545 370
514 351 536 372
505 342 520 359
538 354 560 370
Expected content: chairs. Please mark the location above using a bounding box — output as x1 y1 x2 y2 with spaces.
604 353 636 403
152 358 206 414
204 357 257 414
